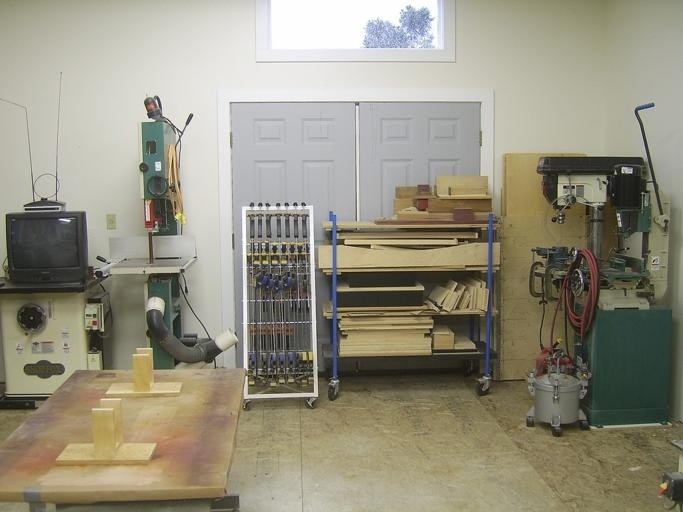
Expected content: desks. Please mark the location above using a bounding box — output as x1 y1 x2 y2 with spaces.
0 370 247 512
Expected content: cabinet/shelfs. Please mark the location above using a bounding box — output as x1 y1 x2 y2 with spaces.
0 283 88 411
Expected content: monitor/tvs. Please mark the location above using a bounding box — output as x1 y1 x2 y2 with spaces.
5 212 88 285
